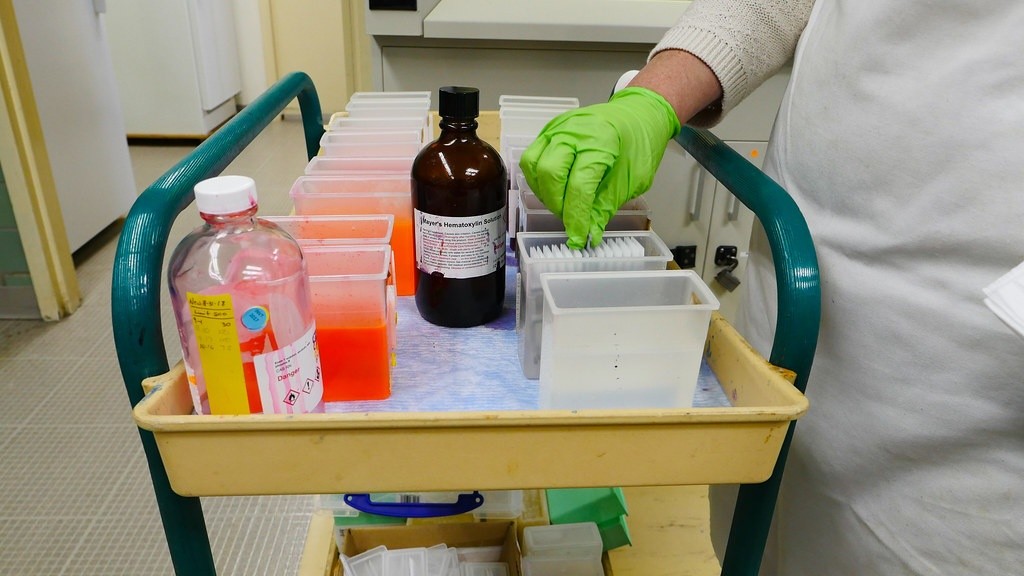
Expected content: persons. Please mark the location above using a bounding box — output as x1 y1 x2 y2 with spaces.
520 1 1023 576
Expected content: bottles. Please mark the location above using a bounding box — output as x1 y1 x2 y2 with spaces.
411 86 509 328
167 175 326 414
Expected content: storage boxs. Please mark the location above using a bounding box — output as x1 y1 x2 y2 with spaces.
224 90 720 411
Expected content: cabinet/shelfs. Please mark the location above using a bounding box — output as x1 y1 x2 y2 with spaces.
110 71 821 576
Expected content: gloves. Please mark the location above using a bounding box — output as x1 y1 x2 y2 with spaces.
518 86 681 248
